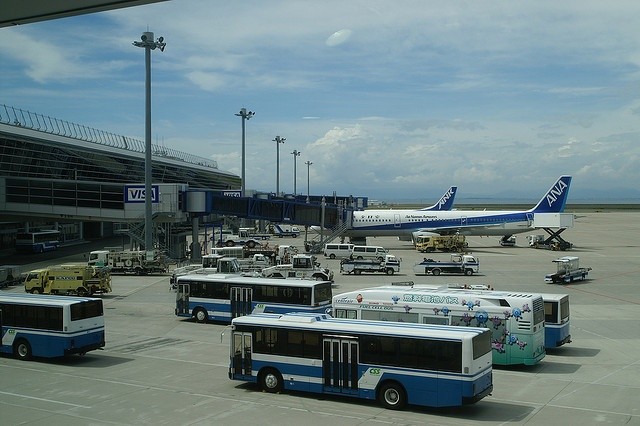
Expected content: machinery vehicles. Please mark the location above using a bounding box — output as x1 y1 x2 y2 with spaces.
526 212 575 250
545 257 592 285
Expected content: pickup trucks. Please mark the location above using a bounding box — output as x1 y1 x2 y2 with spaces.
178 258 260 279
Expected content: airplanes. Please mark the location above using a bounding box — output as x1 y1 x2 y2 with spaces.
309 175 573 246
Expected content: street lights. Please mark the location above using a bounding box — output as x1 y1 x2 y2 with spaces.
131 23 166 250
233 105 256 197
305 160 314 196
290 149 301 195
272 135 286 198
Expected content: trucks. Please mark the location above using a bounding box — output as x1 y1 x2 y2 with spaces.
417 234 467 254
417 255 480 276
201 253 271 268
23 262 113 298
0 265 27 289
238 227 273 239
260 253 334 284
211 244 298 265
273 224 300 239
224 228 261 247
341 255 401 276
87 249 170 276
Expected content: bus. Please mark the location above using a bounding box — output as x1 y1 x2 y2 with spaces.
461 286 571 348
16 230 61 253
331 288 546 367
170 271 333 324
0 294 105 364
229 311 494 413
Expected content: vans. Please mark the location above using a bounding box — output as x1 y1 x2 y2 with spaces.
209 229 232 242
324 242 355 258
351 246 389 258
